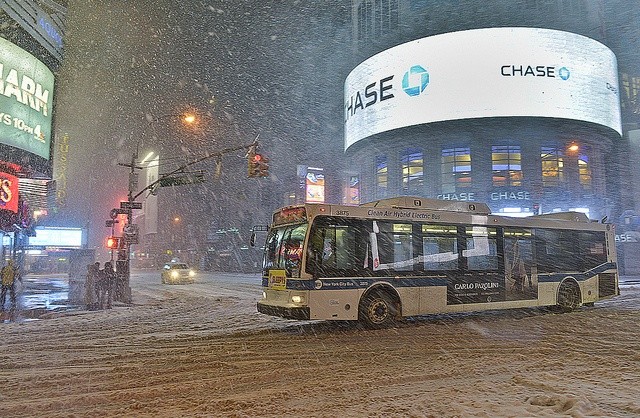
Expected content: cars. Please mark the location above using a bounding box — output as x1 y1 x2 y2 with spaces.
160 262 194 285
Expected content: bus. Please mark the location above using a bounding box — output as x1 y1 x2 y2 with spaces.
251 196 620 329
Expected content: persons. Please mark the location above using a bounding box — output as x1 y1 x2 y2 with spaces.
92 261 102 303
0 259 23 309
98 262 117 310
85 262 96 310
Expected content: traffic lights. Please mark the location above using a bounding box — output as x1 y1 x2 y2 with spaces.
248 154 261 178
108 238 118 248
261 158 269 176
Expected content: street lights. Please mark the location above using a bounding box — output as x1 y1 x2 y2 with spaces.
123 114 194 301
110 209 116 265
